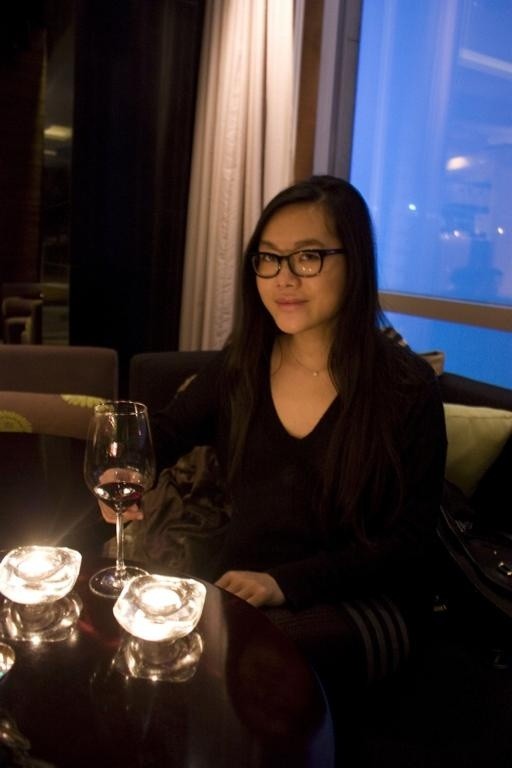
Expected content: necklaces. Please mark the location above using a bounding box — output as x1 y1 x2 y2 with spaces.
286 336 328 377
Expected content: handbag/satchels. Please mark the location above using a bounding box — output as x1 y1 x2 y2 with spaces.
432 502 512 615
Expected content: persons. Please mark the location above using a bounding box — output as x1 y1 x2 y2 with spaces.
97 175 451 682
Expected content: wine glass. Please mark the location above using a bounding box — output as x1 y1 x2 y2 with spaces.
81 399 156 596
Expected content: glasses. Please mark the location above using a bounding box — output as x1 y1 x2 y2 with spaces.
250 248 345 279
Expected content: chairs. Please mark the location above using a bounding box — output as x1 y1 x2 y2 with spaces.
1 342 228 441
